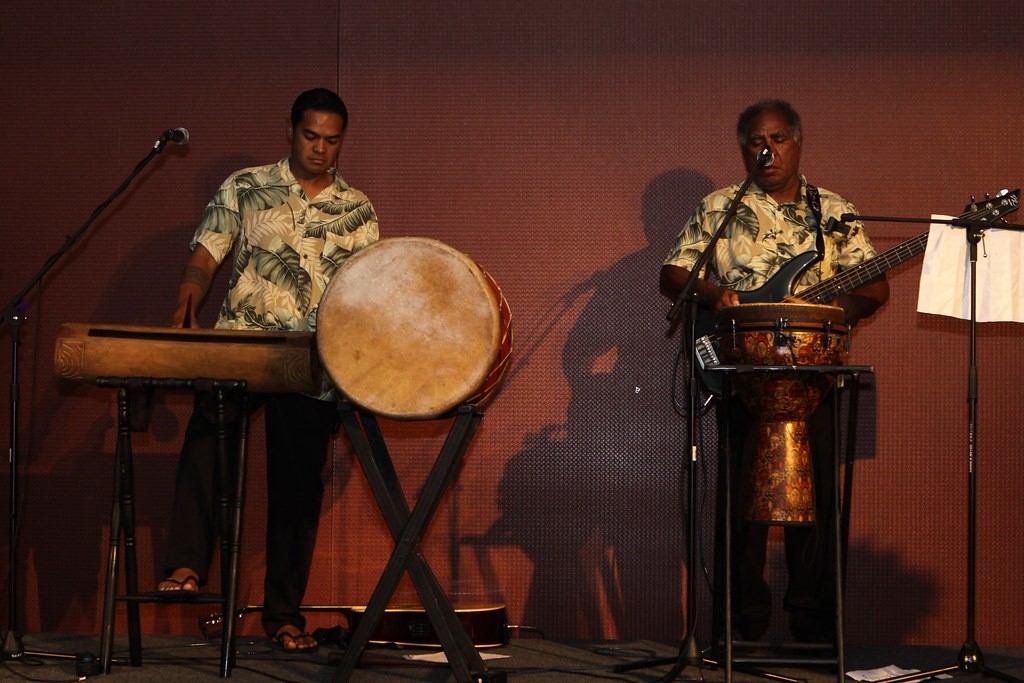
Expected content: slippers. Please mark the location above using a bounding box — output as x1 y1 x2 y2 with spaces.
157 575 200 595
271 631 319 655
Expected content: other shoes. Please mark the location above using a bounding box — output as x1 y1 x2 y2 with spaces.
716 628 751 652
791 608 835 643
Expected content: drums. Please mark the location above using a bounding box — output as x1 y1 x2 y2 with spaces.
314 233 517 421
715 302 849 531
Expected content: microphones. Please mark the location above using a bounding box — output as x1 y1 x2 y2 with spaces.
167 127 190 144
757 145 775 167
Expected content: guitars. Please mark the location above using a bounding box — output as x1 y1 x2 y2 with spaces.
198 601 508 649
678 188 1022 408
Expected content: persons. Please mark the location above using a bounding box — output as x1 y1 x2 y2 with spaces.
157 88 380 652
659 101 890 656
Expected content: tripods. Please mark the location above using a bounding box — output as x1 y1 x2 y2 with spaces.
860 229 1024 683
612 164 807 683
